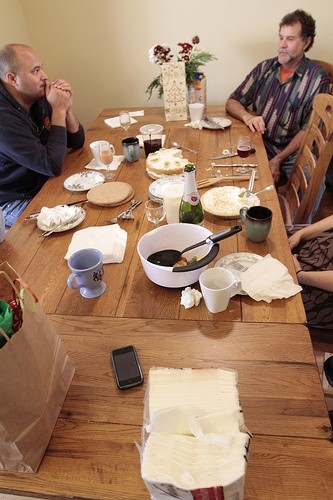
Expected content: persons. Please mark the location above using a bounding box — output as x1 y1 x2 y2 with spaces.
226 9 330 224
289 216 333 330
0 43 85 227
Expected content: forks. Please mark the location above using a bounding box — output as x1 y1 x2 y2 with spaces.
105 198 143 224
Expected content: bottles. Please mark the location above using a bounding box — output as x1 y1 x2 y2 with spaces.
179 164 205 227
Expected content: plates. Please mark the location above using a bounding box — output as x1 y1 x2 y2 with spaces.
201 117 232 129
63 170 106 192
215 253 263 296
37 207 86 232
199 185 260 219
149 177 186 205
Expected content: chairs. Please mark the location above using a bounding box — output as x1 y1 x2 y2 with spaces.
276 61 333 236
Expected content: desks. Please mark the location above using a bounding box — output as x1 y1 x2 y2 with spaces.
0 107 333 500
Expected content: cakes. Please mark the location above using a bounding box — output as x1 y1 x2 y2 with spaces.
145 147 190 180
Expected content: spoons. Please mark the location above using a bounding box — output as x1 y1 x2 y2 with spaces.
145 225 242 267
171 141 200 155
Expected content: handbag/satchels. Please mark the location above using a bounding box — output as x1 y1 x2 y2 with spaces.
0 261 77 474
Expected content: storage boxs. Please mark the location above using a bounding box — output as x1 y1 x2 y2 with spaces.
161 61 189 121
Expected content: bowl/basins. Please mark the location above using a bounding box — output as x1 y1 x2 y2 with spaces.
138 223 219 288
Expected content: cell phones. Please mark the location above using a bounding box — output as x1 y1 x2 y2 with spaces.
111 345 143 390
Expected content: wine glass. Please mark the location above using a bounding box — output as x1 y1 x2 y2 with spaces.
119 111 131 137
99 145 116 183
236 136 252 173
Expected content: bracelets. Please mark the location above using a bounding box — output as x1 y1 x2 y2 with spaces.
296 270 304 274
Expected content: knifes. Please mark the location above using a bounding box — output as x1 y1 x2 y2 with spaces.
208 153 258 167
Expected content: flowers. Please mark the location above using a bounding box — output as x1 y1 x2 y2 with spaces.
144 35 218 100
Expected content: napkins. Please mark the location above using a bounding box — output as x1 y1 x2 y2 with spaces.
62 223 128 264
240 253 302 304
140 366 251 500
84 154 125 171
104 114 138 129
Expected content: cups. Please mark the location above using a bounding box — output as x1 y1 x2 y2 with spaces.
239 206 273 243
199 267 241 314
146 198 166 223
163 180 185 224
188 103 205 124
121 124 164 164
67 249 106 299
90 141 116 166
